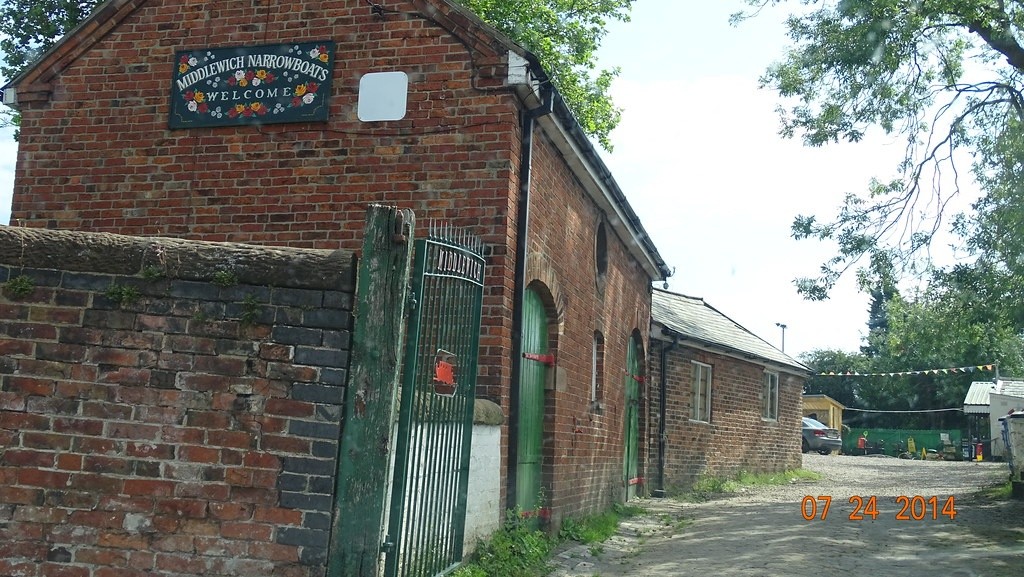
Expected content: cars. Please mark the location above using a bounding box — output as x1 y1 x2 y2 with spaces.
802 417 842 455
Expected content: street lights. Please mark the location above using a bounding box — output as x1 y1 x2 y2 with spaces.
775 322 788 353
